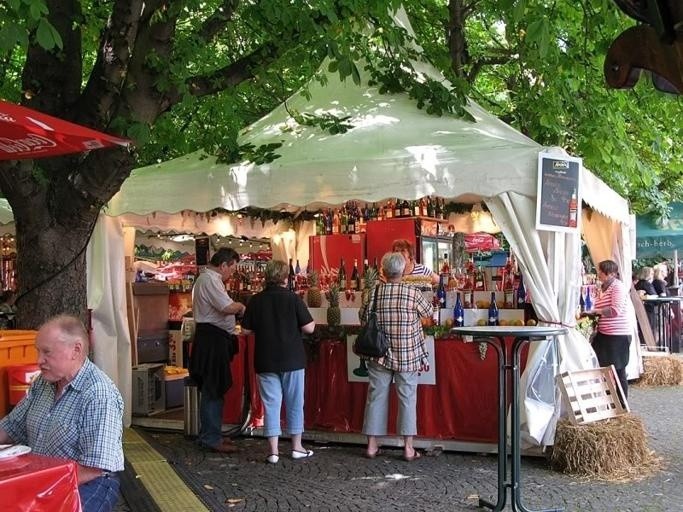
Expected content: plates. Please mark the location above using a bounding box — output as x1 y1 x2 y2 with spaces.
0 443 32 459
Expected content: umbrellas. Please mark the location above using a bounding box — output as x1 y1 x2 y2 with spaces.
0 100 133 162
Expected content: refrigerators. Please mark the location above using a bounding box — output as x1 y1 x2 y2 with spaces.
367 216 452 285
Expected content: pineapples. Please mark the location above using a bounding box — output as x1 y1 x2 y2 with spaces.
326 285 343 325
361 264 379 307
307 268 322 308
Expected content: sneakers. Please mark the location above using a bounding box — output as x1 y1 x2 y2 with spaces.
207 437 238 453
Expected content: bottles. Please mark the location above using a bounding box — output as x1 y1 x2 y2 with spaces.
440 261 493 294
314 196 448 235
488 291 499 327
452 292 466 327
502 276 515 307
517 275 526 307
338 259 348 292
210 264 270 295
569 193 577 227
284 258 318 293
584 287 593 309
348 255 387 293
426 274 446 328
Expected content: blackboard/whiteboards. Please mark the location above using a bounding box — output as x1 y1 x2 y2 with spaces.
535 152 583 234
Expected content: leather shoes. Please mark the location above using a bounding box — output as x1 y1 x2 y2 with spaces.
291 449 314 459
266 454 279 464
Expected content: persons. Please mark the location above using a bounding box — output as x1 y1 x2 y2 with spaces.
0 311 126 512
240 259 316 465
392 238 440 284
356 251 433 460
634 259 677 354
188 246 246 452
582 259 634 409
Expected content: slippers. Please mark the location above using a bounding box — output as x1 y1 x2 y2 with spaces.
403 450 423 461
367 448 386 458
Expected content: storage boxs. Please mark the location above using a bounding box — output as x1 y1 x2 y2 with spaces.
165 375 185 409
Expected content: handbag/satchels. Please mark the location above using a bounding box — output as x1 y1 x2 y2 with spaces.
352 313 389 357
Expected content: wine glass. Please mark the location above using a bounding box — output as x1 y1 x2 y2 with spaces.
321 290 356 308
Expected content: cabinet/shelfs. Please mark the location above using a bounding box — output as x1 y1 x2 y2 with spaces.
186 274 534 447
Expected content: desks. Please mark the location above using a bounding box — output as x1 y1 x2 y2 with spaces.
450 325 569 512
643 296 680 350
